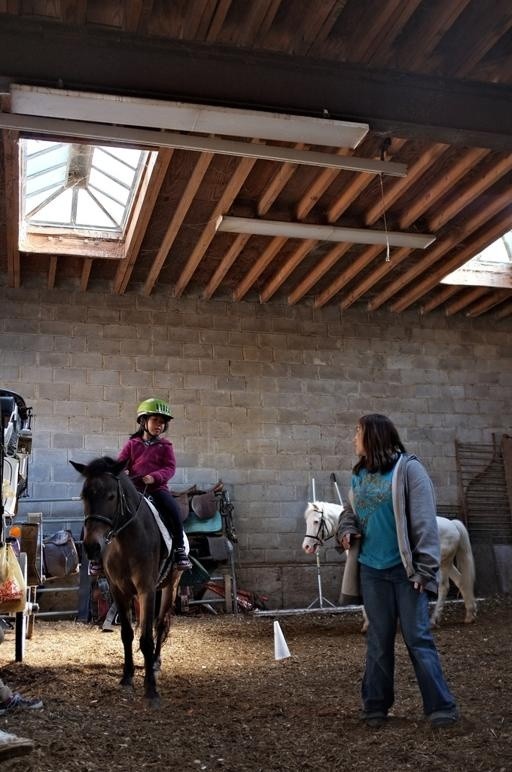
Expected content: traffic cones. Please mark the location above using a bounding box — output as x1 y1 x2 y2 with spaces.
274 621 291 660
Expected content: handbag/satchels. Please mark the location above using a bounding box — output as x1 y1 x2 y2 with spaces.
44 529 80 578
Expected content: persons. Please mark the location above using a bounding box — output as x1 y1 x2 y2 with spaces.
0 628 42 715
91 398 192 575
337 414 458 729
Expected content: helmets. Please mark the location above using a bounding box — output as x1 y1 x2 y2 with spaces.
136 398 174 424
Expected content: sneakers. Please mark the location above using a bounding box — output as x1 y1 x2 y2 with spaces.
1 691 42 715
90 561 103 576
0 730 35 762
428 694 461 728
173 547 192 571
365 709 387 730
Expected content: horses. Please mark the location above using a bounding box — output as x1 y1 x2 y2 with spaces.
68 457 183 711
302 500 478 632
